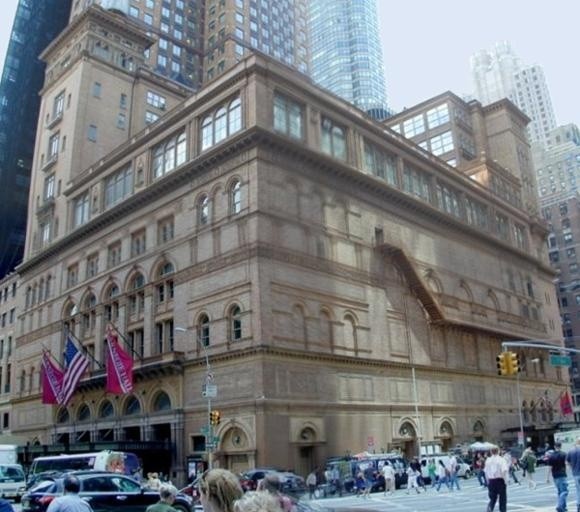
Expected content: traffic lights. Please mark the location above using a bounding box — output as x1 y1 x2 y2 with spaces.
209 412 215 427
496 354 505 377
216 416 220 425
511 352 522 377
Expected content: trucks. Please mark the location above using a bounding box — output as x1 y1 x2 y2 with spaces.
0 443 26 504
552 428 580 453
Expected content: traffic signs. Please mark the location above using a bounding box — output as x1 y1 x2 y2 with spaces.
550 353 573 368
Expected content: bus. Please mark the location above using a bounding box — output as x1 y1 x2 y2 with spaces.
26 449 142 483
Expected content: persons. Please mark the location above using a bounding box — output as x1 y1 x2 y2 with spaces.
46 476 94 512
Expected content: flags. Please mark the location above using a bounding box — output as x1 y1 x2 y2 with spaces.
560 389 573 417
58 334 91 407
542 389 550 412
105 325 134 394
41 349 64 405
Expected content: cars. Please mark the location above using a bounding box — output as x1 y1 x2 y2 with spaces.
533 450 550 466
23 468 308 512
324 438 526 501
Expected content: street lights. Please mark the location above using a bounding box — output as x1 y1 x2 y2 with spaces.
517 357 540 448
174 326 215 471
